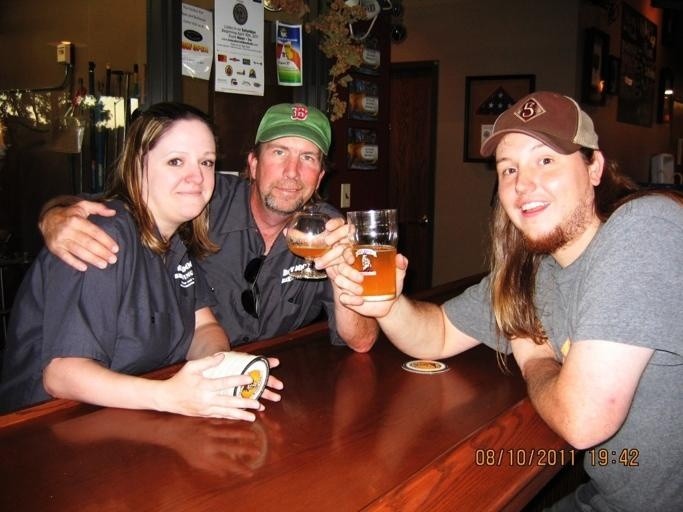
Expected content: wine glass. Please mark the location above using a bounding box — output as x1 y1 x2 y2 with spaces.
284 212 331 281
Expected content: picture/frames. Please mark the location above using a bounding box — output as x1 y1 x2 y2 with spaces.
608 54 623 98
462 75 535 161
574 27 609 109
616 4 659 128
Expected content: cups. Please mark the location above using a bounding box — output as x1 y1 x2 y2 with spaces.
345 208 400 303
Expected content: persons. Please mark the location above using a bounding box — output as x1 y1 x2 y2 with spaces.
37 102 382 355
333 91 682 511
1 100 285 422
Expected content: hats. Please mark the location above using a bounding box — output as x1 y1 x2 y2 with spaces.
478 84 598 163
251 100 332 156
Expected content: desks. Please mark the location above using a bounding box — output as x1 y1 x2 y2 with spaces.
0 272 575 511
